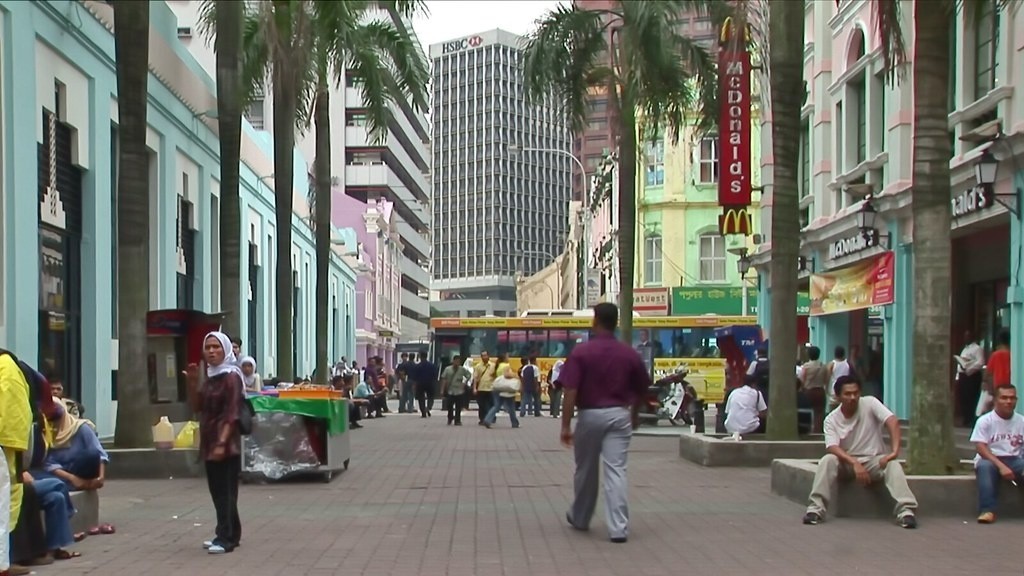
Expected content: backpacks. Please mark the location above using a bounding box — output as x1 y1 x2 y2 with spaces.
755 359 770 385
523 366 534 384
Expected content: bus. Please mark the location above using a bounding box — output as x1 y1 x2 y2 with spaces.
428 315 759 411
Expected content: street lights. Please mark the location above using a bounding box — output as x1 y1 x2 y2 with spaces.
507 144 588 318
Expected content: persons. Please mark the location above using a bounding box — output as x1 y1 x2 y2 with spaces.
482 354 524 429
0 347 110 576
517 358 533 417
314 350 438 428
723 378 767 435
240 356 265 393
442 355 471 425
970 383 1024 522
559 303 649 543
987 328 1011 410
801 375 920 528
232 337 243 362
637 329 659 358
521 357 543 417
548 360 562 418
201 332 245 555
473 349 498 424
745 339 868 433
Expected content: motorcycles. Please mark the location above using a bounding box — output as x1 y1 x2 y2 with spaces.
633 369 698 426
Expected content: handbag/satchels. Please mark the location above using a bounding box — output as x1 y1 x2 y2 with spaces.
974 389 994 417
493 375 521 397
237 393 257 434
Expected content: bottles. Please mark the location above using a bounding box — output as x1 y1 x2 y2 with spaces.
155 416 174 450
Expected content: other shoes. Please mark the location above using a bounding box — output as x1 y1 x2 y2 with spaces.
203 541 226 553
72 531 87 542
422 410 430 417
54 548 81 558
8 563 29 576
384 408 387 412
350 423 362 429
977 512 998 523
448 417 461 425
368 414 384 418
23 554 53 565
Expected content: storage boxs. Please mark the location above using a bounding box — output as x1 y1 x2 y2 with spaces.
278 390 343 399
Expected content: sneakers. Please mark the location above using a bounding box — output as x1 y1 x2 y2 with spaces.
897 516 916 528
803 512 822 524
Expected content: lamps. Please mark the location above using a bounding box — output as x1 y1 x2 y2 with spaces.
843 183 874 200
974 149 1021 219
737 249 761 291
857 201 891 251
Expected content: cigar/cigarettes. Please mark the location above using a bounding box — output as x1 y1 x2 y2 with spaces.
1012 481 1017 486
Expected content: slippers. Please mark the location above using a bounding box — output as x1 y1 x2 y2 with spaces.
89 522 115 534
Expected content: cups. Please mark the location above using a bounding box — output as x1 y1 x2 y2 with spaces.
689 424 696 435
734 432 739 441
